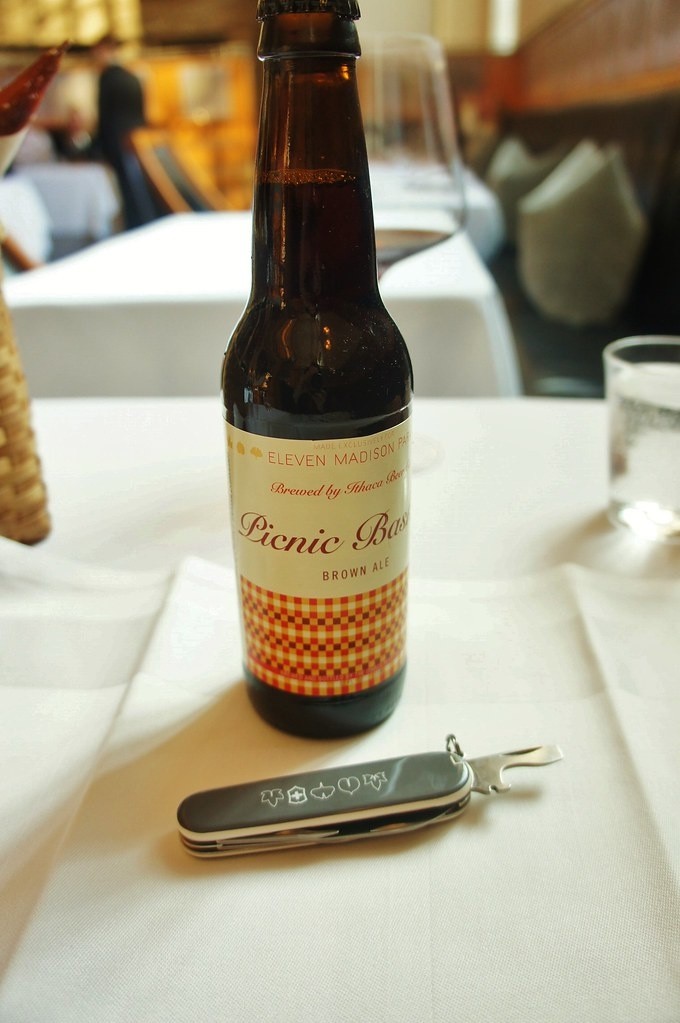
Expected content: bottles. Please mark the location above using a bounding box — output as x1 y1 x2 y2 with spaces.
220 0 412 741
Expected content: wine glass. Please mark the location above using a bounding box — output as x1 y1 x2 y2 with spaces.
354 28 468 284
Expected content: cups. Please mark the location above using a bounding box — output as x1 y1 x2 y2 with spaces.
602 334 680 542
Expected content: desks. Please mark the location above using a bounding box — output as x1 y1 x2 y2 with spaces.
1 162 680 1023
